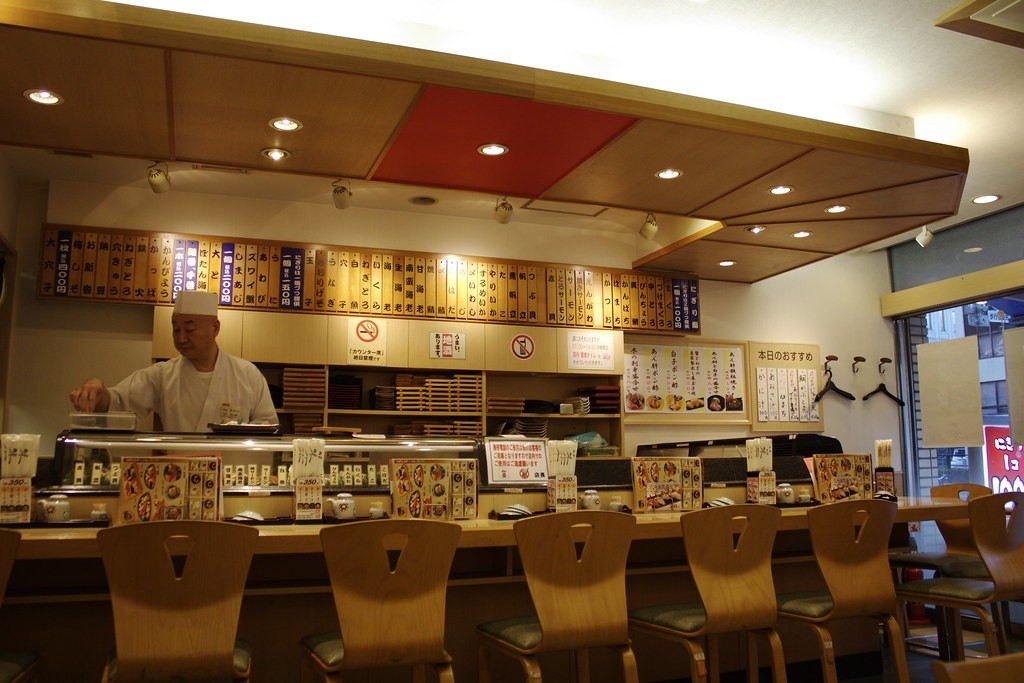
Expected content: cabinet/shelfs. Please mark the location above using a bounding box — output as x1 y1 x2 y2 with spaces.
149 306 629 460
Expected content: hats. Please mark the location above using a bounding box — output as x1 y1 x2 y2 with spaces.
173 291 218 316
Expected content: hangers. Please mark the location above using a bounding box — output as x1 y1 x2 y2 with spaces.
862 373 905 406
815 370 856 402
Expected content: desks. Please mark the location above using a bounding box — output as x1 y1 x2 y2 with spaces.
0 495 1013 604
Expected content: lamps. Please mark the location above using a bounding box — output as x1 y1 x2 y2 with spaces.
916 225 933 248
640 212 659 240
495 195 513 225
331 179 353 210
146 161 172 194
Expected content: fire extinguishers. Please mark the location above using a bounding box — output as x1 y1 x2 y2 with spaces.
901 567 926 620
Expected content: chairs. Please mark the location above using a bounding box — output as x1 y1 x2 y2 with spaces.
98 521 254 683
476 509 639 683
888 483 1024 683
778 499 910 683
0 529 40 683
300 518 461 683
628 503 789 683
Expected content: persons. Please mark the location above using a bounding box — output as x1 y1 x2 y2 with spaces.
67 290 279 434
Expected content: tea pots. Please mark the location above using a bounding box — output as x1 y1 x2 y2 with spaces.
580 489 602 511
326 493 356 521
38 494 70 523
776 482 795 503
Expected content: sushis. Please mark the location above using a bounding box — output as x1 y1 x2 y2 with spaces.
830 484 859 498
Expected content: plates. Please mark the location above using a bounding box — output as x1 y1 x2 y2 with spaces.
514 419 548 437
821 457 856 500
666 396 685 412
710 497 736 507
414 465 424 487
873 491 892 499
233 511 265 521
626 394 646 411
686 397 705 410
566 397 591 414
328 383 361 408
409 490 421 517
646 395 665 411
500 504 532 517
434 484 444 496
707 395 725 411
636 460 679 510
121 456 183 521
430 465 445 479
